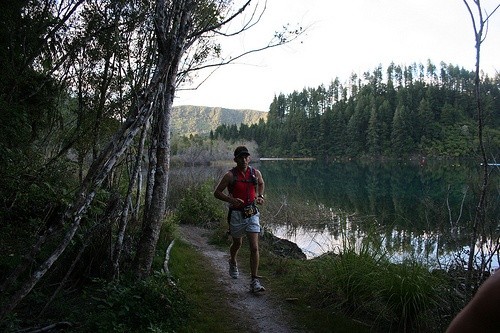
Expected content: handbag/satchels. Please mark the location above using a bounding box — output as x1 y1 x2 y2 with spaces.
242 203 257 219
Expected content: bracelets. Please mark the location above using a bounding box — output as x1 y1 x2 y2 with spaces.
258 194 264 199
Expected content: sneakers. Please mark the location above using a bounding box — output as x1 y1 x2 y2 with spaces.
250 278 265 292
229 259 239 278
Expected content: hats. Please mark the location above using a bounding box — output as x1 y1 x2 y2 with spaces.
234 146 250 157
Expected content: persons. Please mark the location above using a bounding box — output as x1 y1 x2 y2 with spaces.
213 146 266 294
446 269 500 333
